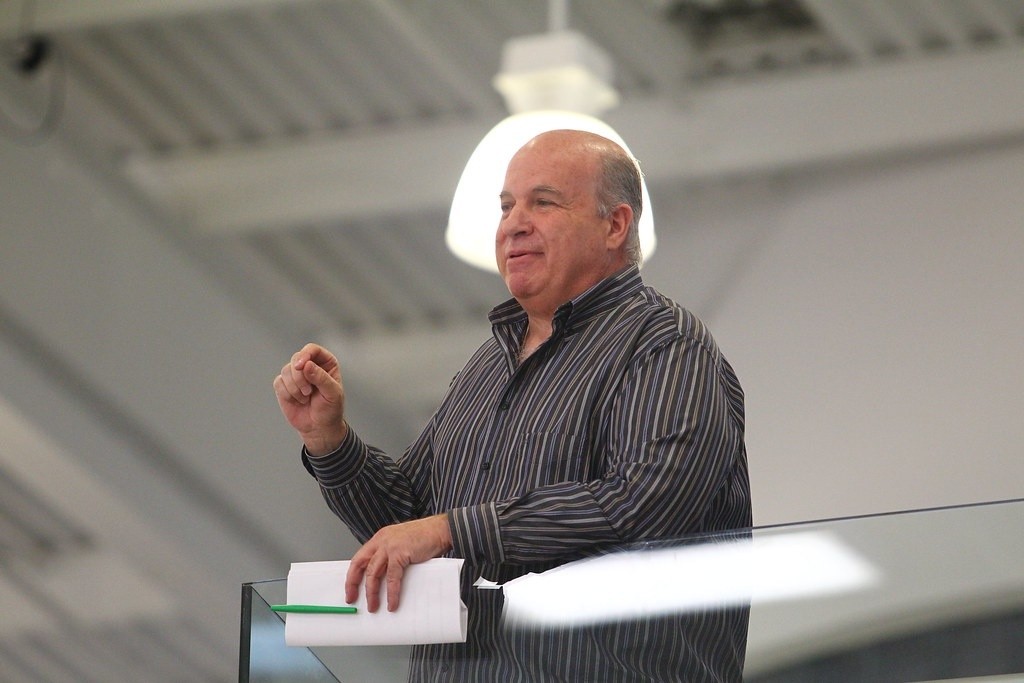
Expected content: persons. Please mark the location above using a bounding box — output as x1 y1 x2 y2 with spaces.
272 130 754 682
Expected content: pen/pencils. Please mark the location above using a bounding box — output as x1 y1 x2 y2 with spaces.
270 604 357 615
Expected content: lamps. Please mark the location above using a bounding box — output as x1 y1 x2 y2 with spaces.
444 0 656 274
